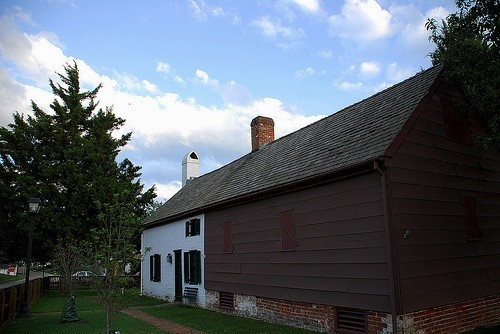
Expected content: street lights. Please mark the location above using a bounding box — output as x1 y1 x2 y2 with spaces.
18 196 42 315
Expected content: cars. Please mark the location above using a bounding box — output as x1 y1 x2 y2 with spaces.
72 270 98 276
